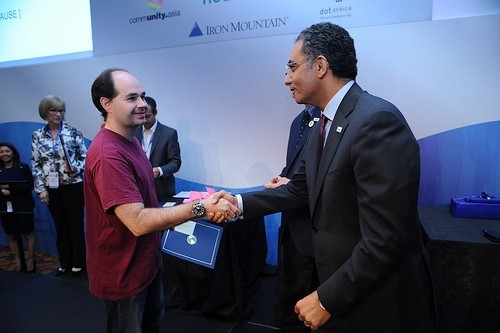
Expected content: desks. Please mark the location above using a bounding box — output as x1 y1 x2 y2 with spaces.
417 206 500 333
160 201 268 324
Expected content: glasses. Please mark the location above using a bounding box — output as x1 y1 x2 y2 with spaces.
49 108 65 114
285 55 309 73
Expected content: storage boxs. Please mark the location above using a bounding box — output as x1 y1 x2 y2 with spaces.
450 199 500 220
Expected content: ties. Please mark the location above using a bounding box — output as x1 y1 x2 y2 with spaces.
296 109 310 148
317 114 328 163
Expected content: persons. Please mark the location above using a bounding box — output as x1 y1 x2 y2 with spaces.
84 68 241 333
197 21 438 333
134 96 182 203
275 103 321 333
30 95 88 277
0 142 37 274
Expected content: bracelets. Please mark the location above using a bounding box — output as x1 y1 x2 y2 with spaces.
319 301 327 310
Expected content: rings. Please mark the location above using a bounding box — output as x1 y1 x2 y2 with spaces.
304 321 311 327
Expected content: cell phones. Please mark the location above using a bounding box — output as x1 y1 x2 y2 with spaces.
482 229 500 240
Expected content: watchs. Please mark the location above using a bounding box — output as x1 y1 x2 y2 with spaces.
192 199 205 219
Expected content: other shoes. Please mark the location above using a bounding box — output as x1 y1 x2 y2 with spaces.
55 267 66 276
22 258 37 273
70 267 82 277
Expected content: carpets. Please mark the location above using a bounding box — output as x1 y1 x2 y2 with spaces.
0 269 282 333
